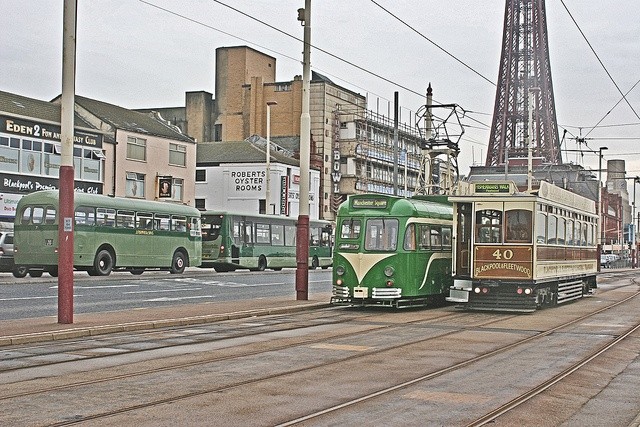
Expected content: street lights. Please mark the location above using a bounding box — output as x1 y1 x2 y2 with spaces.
266 101 277 214
528 88 541 190
633 176 640 268
598 147 608 252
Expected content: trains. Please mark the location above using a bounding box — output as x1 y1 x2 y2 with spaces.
328 193 453 309
445 180 601 312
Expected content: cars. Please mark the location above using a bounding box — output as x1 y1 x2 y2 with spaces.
601 254 609 268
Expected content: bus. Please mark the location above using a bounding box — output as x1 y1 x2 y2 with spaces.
195 208 332 272
14 190 205 276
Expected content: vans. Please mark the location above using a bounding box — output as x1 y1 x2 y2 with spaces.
0 232 43 278
